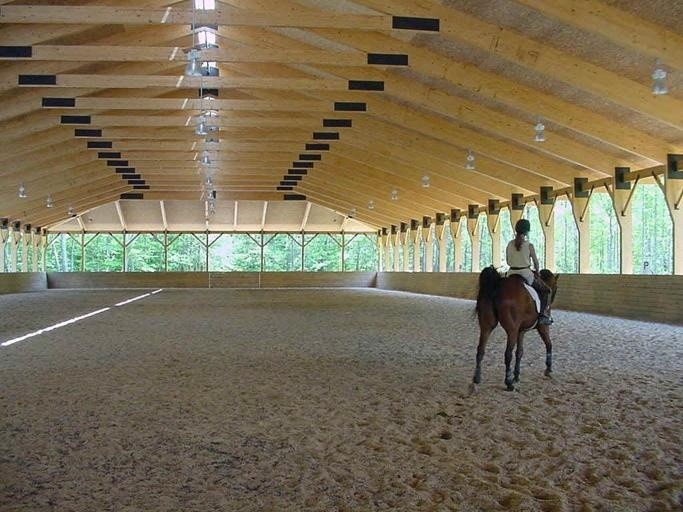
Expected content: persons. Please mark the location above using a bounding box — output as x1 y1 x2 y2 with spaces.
505 219 554 325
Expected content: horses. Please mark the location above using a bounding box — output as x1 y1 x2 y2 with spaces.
462 264 560 396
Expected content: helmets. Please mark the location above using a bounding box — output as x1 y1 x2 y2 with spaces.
515 219 530 234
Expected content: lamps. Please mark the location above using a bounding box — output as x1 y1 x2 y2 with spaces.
651 58 669 95
533 116 546 143
183 0 212 138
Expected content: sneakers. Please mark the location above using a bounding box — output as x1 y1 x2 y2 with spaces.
538 317 553 324
